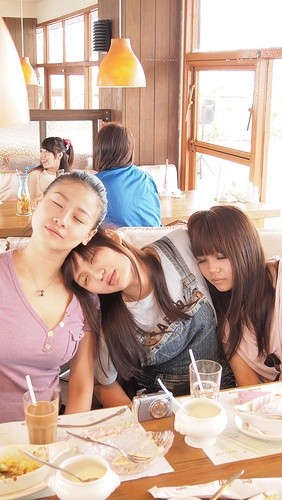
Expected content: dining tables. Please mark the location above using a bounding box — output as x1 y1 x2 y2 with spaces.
0 381 282 500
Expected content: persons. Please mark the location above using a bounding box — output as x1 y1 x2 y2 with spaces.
62 227 236 408
188 205 282 386
27 137 74 215
0 170 107 425
93 124 165 227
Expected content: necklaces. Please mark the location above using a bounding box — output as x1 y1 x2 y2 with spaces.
23 247 61 297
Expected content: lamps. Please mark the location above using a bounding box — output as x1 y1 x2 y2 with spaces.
19 0 37 86
97 0 146 88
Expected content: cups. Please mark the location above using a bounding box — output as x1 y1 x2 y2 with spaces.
173 398 227 448
22 389 60 445
16 173 32 216
188 359 222 398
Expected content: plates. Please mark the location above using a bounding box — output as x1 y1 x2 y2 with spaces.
0 479 48 500
235 415 282 442
0 442 69 496
235 391 282 433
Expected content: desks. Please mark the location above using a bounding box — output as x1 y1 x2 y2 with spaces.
0 190 280 238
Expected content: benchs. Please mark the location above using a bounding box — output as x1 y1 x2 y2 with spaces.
0 164 179 195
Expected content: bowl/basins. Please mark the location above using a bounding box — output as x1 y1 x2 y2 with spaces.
90 430 174 476
56 456 114 495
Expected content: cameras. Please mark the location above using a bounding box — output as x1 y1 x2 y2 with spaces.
132 389 173 422
